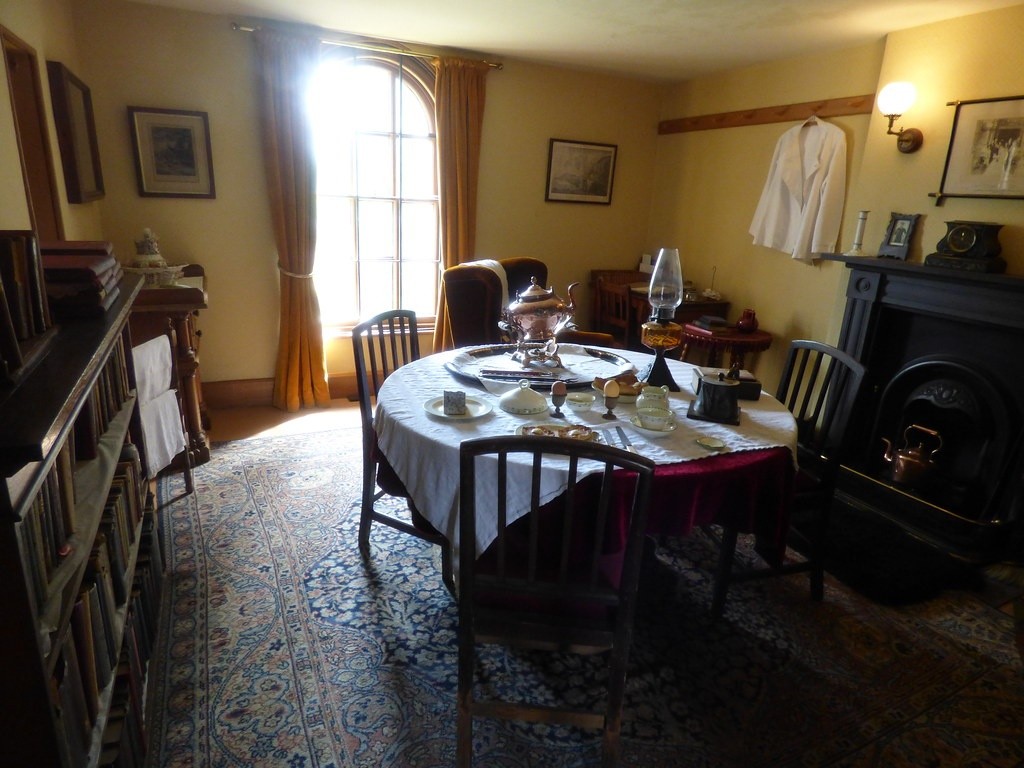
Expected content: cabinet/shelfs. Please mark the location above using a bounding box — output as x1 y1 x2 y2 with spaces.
0 273 165 768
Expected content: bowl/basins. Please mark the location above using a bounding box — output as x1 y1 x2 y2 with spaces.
637 408 676 430
591 382 640 403
121 264 190 287
565 392 596 412
693 371 740 419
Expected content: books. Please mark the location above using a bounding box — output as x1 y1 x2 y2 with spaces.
21 334 168 768
0 229 125 368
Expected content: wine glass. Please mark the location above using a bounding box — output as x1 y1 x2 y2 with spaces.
550 392 567 418
602 394 619 420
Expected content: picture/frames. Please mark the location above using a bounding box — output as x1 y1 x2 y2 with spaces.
47 60 105 205
876 211 922 262
926 95 1024 206
126 104 217 199
544 138 618 205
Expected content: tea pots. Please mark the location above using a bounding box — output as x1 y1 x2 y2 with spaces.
508 276 580 338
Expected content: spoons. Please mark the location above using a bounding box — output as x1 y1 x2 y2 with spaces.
591 432 602 442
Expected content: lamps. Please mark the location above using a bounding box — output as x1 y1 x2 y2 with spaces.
877 81 924 156
639 247 684 393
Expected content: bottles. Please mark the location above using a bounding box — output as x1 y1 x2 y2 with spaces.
129 228 168 268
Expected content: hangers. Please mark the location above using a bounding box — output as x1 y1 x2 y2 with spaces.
802 106 818 127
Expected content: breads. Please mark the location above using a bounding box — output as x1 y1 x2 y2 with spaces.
593 376 650 396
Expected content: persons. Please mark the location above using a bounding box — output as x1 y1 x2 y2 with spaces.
983 136 1021 175
894 222 907 243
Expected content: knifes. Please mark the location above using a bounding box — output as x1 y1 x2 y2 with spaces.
616 426 637 454
602 429 617 448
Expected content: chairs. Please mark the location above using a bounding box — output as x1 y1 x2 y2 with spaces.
595 276 633 350
455 435 658 768
443 257 615 348
351 310 454 586
698 339 873 618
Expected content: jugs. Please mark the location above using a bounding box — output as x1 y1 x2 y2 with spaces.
736 308 759 332
636 385 671 408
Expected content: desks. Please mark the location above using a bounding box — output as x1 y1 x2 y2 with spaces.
377 340 799 623
679 322 773 370
612 282 731 367
126 264 213 464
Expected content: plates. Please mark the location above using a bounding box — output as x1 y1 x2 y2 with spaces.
697 437 727 450
630 416 679 437
514 421 593 442
423 396 493 419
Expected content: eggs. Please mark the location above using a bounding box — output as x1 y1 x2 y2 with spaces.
551 380 566 394
604 380 619 397
558 425 592 441
522 425 554 436
